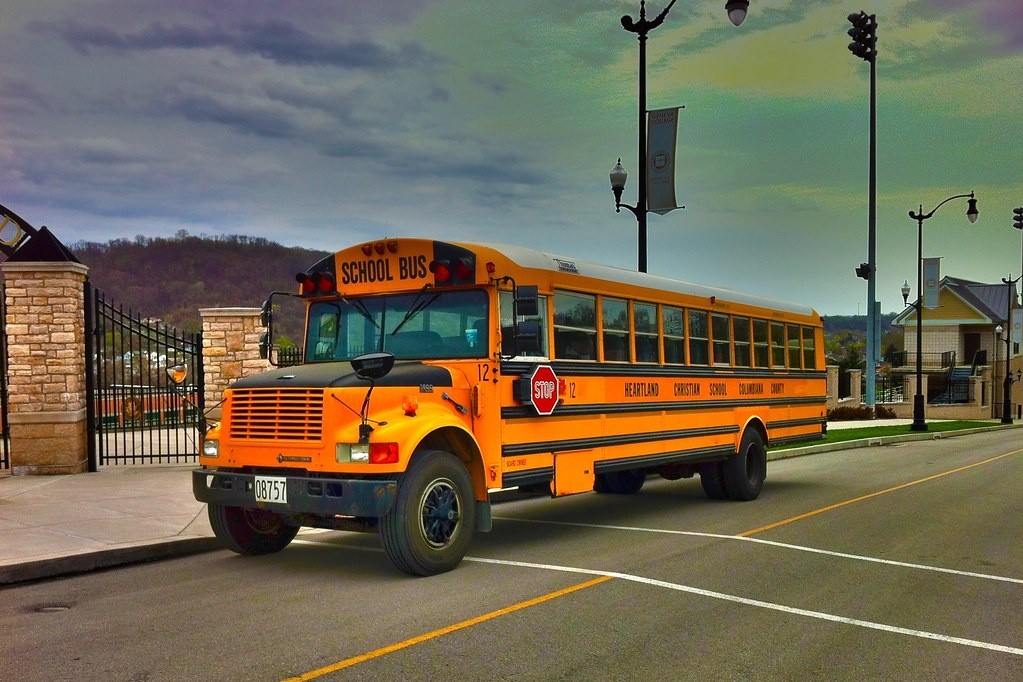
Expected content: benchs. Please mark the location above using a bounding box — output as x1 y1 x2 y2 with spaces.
376 331 444 355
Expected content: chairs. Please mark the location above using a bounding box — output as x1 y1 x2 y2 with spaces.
556 329 814 377
472 319 516 354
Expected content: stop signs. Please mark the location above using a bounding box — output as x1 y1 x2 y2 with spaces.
530 365 560 415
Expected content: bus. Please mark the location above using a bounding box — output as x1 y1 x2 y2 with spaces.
190 239 832 577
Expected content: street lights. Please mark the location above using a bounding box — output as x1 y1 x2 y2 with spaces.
995 273 1023 424
901 190 979 431
848 10 878 422
609 1 750 275
